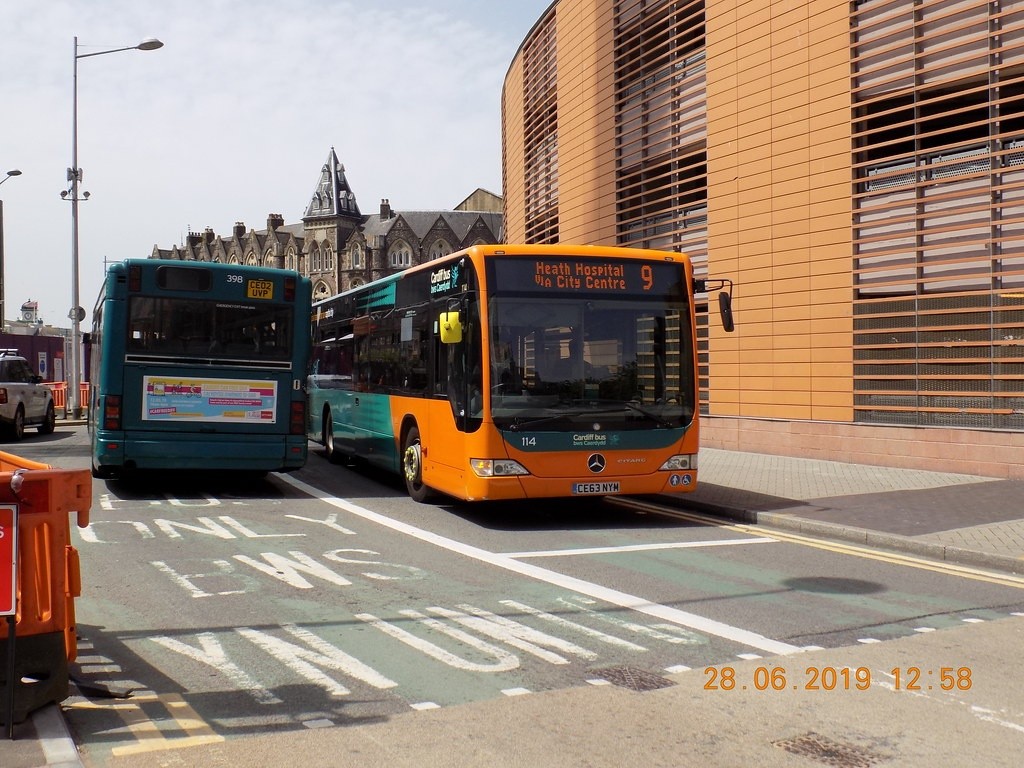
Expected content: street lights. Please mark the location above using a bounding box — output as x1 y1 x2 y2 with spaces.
58 34 164 420
0 167 22 328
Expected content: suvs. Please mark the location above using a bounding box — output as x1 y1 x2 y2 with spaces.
0 348 55 442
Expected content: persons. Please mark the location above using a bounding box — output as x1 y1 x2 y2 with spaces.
545 339 596 398
469 337 523 396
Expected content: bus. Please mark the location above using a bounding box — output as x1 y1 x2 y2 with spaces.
78 257 313 490
308 243 734 508
